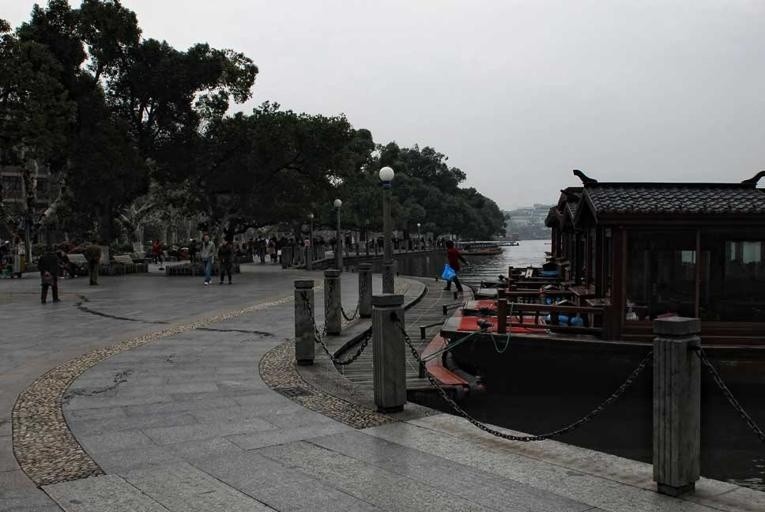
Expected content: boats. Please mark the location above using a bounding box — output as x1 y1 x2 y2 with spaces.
440 164 765 404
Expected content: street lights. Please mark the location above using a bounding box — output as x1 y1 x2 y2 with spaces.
304 211 316 269
332 198 345 272
377 166 395 296
363 218 369 253
415 221 421 251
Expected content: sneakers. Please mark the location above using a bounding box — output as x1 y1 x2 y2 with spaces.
444 287 450 290
219 282 232 285
205 278 213 286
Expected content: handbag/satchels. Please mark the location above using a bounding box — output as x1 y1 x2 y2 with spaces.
41 275 54 286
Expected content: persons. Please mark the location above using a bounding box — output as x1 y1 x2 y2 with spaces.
217 237 232 286
37 243 61 305
56 250 84 278
234 233 444 263
82 239 102 287
442 240 470 292
199 233 217 287
144 239 187 270
187 239 199 264
